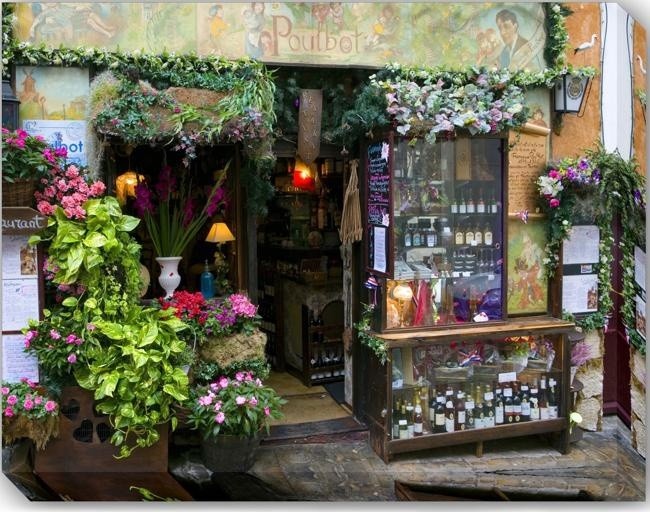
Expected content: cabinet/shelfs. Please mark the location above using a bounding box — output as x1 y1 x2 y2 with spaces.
351 121 577 465
257 147 343 388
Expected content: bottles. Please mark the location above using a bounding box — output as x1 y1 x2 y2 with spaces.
259 183 307 276
449 187 498 214
321 157 343 176
455 222 493 245
401 218 451 248
201 258 215 298
310 345 345 381
310 312 324 342
258 282 276 369
392 375 559 439
309 187 342 230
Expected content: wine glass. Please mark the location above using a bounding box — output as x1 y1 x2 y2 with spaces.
454 247 498 275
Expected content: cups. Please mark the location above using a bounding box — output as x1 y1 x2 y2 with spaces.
432 253 443 272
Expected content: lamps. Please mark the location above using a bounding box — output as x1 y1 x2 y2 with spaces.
204 222 236 253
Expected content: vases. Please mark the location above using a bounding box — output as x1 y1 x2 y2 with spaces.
196 428 261 475
172 401 200 440
153 256 183 299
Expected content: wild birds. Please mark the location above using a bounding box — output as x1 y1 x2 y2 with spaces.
637 54 647 76
573 34 599 67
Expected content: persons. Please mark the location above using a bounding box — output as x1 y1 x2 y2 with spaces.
515 233 544 309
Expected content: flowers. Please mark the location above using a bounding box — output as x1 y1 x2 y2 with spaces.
1 126 107 223
132 165 230 228
197 371 269 423
515 146 647 357
156 291 258 336
2 377 56 418
321 1 598 145
21 324 95 364
